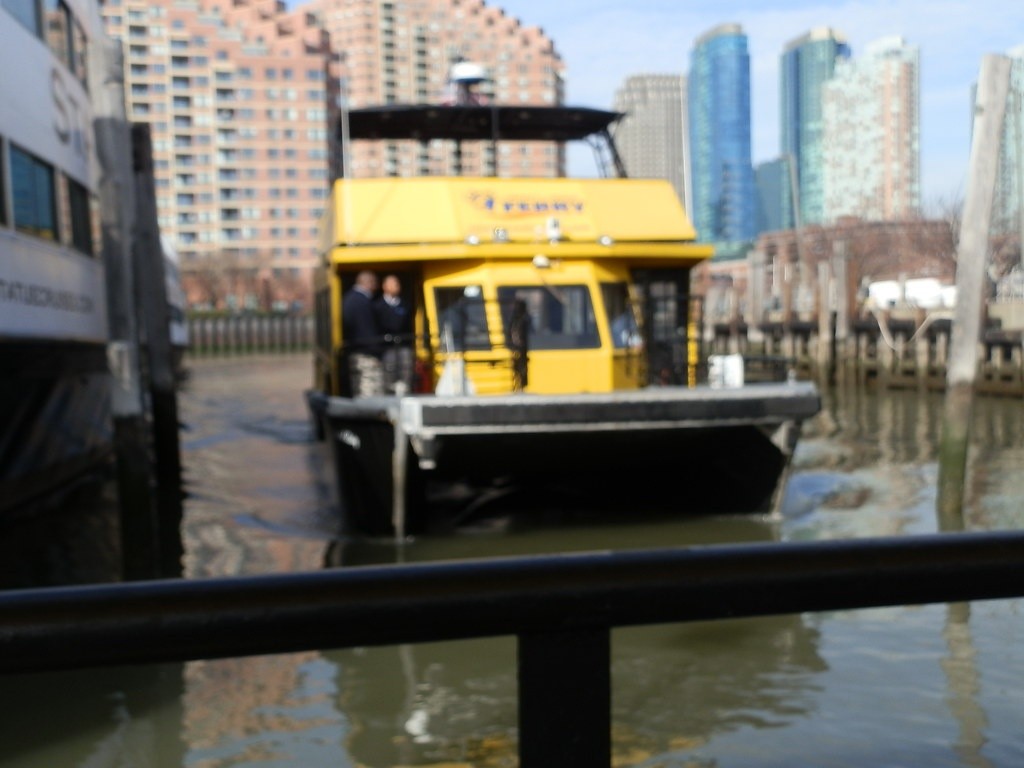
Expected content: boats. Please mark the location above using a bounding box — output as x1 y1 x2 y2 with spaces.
305 61 824 533
0 0 193 594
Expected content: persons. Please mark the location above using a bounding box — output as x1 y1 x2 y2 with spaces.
374 275 415 394
343 271 387 400
